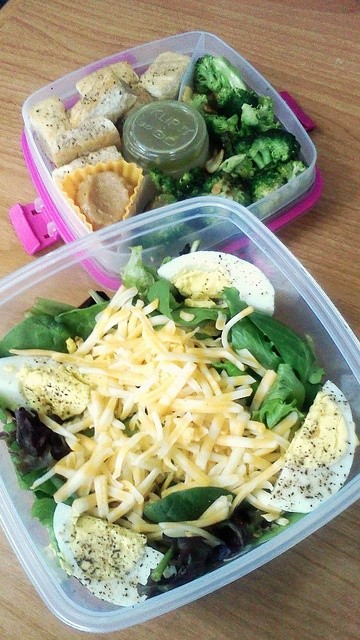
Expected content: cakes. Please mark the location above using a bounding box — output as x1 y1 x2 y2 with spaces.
58 161 144 233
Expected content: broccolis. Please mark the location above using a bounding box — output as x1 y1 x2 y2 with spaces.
144 53 309 246
